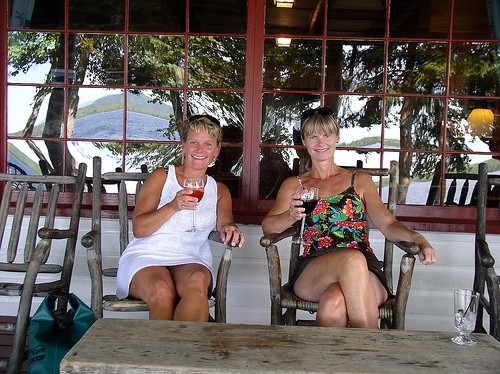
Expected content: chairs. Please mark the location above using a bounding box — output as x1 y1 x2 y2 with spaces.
82 156 241 324
471 162 500 342
260 159 421 330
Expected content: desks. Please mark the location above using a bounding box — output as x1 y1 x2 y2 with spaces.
0 163 87 374
60 319 500 374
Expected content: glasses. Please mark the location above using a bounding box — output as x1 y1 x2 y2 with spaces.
189 115 220 128
301 108 335 127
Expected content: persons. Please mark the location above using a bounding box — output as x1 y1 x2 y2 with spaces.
262 108 437 329
116 115 246 322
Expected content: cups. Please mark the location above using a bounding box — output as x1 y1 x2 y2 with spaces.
451 289 480 346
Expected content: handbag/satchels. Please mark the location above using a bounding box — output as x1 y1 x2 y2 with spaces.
27 289 95 374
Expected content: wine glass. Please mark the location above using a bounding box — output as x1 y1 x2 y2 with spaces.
289 185 320 246
183 179 204 233
